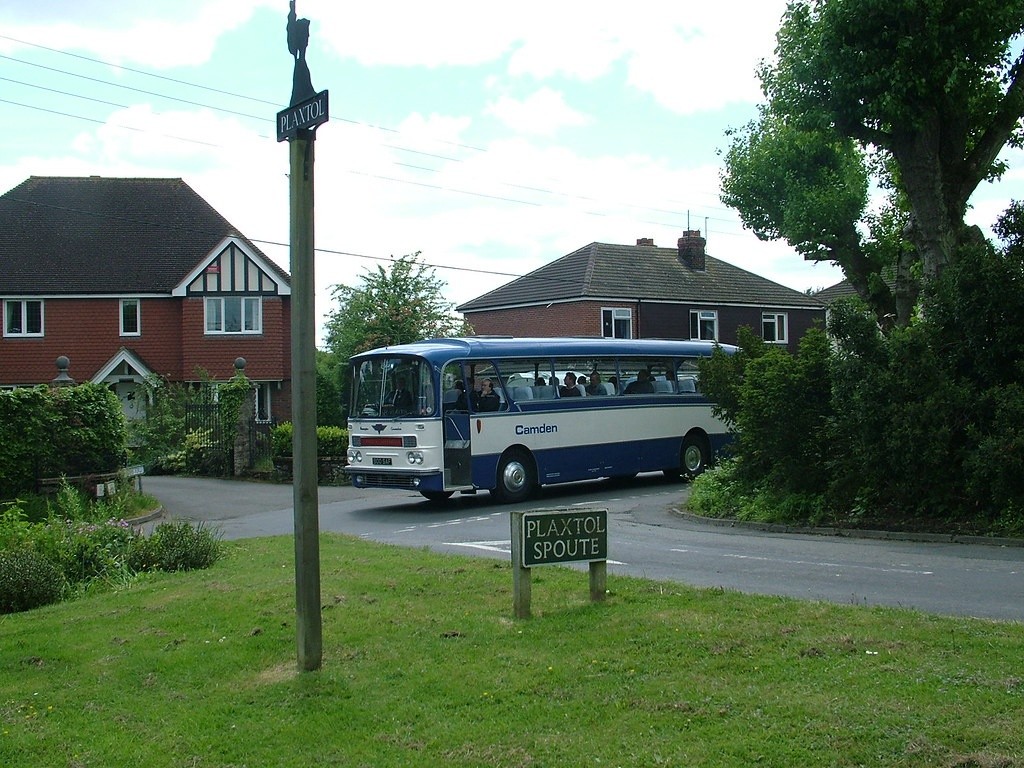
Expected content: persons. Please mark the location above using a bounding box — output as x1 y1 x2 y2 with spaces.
533 368 678 398
453 378 500 414
384 378 412 409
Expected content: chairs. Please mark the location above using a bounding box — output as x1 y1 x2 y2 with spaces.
442 377 696 413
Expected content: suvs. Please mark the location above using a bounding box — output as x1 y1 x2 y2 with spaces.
625 376 695 393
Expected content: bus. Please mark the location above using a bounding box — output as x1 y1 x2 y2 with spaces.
348 336 749 503
